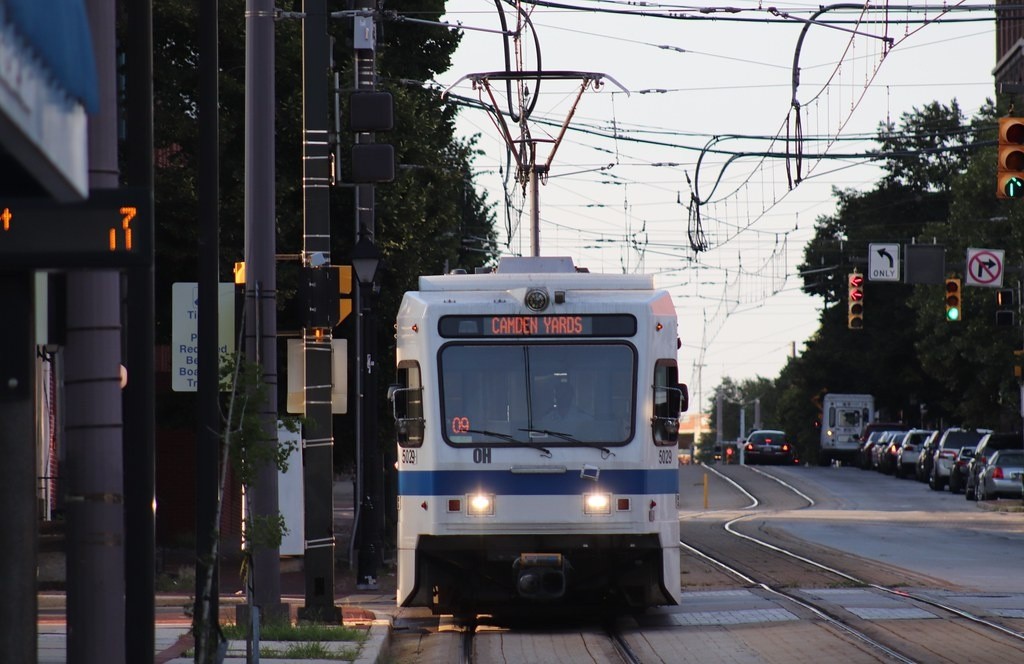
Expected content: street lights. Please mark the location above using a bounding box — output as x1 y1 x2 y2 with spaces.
348 230 387 588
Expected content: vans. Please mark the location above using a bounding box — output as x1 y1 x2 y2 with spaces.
818 393 873 466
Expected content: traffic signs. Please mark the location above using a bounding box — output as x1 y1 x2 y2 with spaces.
869 244 898 282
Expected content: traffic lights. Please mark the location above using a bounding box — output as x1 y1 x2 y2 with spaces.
726 447 734 455
945 279 961 322
714 454 721 460
849 273 868 327
312 266 353 341
2 193 148 271
998 118 1024 200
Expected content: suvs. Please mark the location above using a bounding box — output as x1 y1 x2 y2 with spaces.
895 430 934 480
967 434 1023 499
854 422 901 471
929 430 994 493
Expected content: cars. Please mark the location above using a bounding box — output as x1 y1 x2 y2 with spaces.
861 430 908 474
743 430 800 465
979 450 1023 499
916 430 952 480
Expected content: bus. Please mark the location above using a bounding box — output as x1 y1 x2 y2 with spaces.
387 255 687 629
